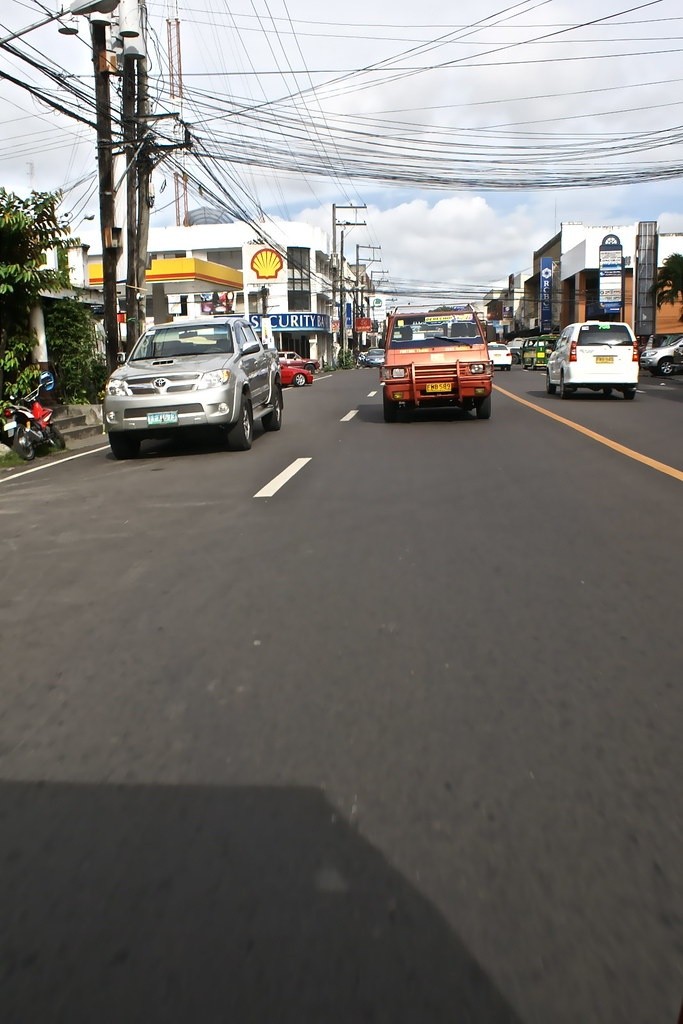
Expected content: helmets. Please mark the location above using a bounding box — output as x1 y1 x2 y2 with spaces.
39 371 54 391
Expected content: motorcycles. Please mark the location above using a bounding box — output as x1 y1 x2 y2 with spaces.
4 373 66 461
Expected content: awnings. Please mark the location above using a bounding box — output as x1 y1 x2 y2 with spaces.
506 325 559 340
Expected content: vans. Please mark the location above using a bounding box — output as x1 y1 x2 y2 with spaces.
645 332 683 350
518 333 559 371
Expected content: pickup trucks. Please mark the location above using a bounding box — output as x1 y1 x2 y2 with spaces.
102 316 284 463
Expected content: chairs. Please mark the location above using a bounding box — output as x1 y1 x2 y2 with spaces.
451 323 470 337
392 326 413 340
216 339 231 351
161 341 182 355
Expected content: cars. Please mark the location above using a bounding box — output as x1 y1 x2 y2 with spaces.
546 322 641 401
277 361 314 388
487 342 512 371
362 349 386 368
641 334 683 377
276 352 320 375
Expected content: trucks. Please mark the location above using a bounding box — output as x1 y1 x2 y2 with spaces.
381 304 496 422
